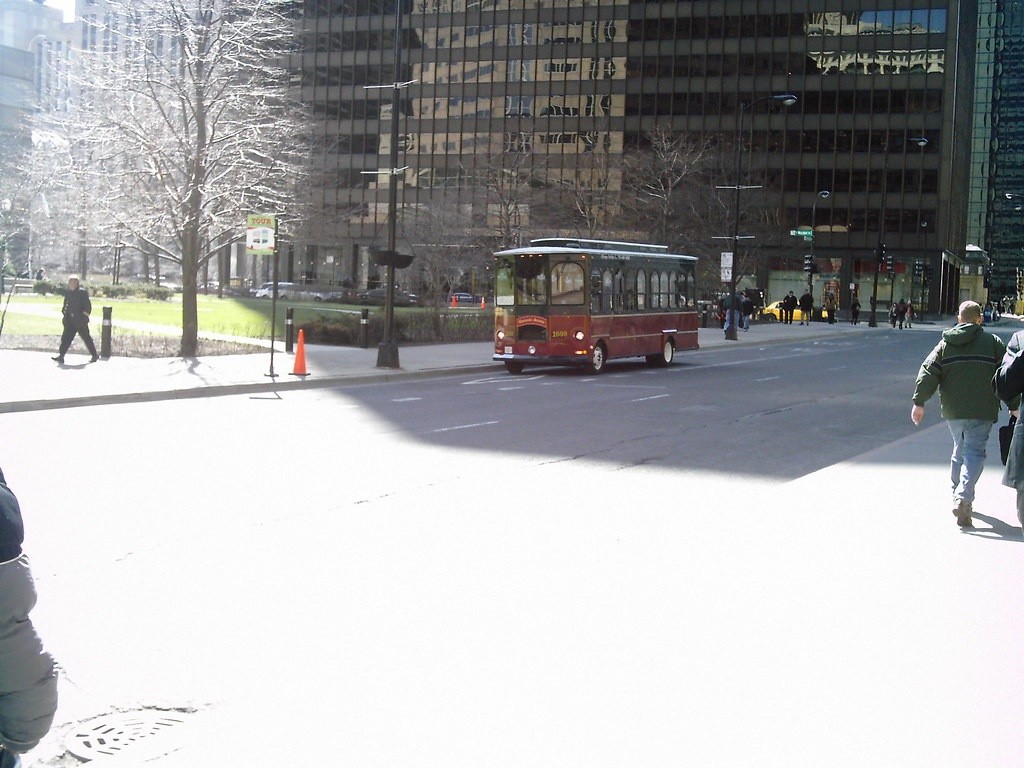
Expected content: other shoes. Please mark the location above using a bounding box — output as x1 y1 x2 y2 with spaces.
89 354 99 363
51 355 64 363
953 499 972 527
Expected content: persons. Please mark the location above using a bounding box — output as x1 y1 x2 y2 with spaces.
991 329 1024 540
911 301 1019 526
895 299 908 329
850 299 861 326
51 277 99 363
997 301 1001 317
979 303 983 311
825 293 838 324
0 467 60 768
905 301 914 328
799 289 813 326
1010 303 1015 315
717 288 753 339
783 291 797 324
888 303 898 328
591 282 612 310
36 270 43 280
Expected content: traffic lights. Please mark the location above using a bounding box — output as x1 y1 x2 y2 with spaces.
886 254 893 274
915 259 925 276
804 254 813 272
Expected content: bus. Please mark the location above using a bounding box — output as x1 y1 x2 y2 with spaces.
489 238 700 376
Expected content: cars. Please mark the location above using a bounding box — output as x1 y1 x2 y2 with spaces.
696 291 766 327
148 268 362 306
359 286 421 307
762 300 829 322
449 292 494 303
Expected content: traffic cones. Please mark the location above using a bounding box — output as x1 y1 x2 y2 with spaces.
288 329 311 376
451 293 459 309
480 295 487 309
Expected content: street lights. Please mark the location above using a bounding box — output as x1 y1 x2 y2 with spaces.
808 191 830 321
725 95 799 341
869 138 929 328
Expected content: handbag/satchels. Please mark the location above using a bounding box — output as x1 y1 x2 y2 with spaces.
999 415 1017 465
